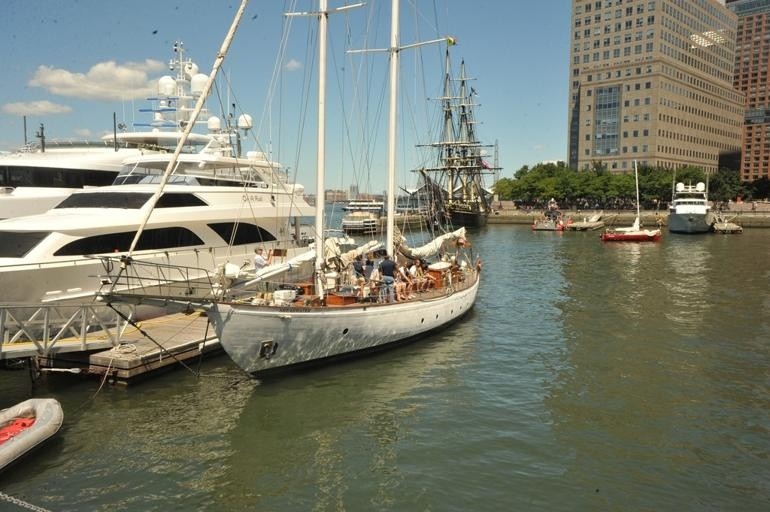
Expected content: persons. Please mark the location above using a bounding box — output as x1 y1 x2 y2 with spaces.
354 254 436 305
254 247 272 273
341 234 355 244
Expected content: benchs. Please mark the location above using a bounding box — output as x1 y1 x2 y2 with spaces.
289 263 465 308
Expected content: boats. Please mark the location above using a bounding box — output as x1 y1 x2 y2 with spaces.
0 39 317 336
667 172 715 235
0 396 66 470
598 229 664 242
531 209 573 232
